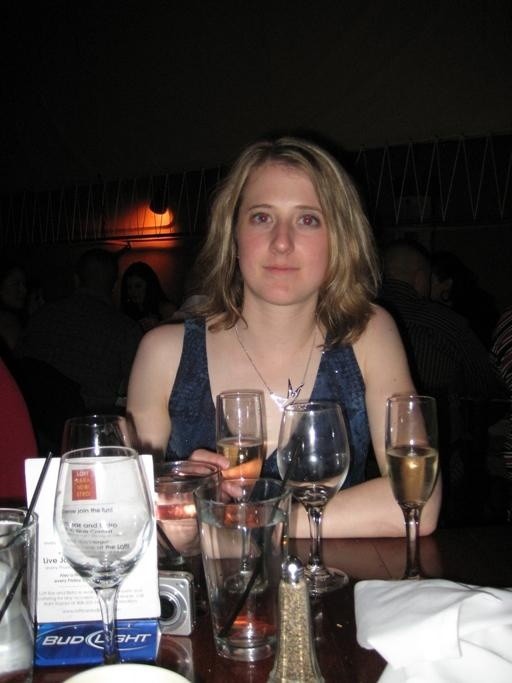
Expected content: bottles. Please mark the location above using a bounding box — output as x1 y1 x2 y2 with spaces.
264 556 328 682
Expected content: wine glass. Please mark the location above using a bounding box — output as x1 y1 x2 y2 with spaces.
273 402 352 599
61 412 139 456
55 443 154 666
155 456 203 631
213 387 268 596
383 393 440 582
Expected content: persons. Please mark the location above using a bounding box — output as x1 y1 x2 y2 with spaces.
291 536 447 584
380 242 512 548
2 248 181 508
112 132 447 558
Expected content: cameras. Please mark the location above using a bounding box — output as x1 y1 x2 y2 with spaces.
159 568 198 637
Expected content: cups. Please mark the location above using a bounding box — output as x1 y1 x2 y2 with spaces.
0 506 37 683
195 476 293 661
